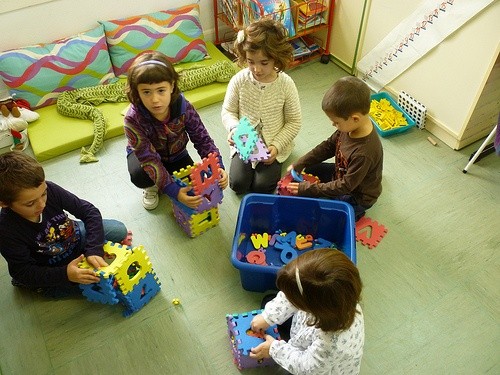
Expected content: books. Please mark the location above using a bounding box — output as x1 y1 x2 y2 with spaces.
221 0 328 61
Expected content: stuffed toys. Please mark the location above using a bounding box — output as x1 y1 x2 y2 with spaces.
57 61 238 164
0 96 40 132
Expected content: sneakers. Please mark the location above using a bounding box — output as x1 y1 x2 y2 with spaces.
140 184 160 211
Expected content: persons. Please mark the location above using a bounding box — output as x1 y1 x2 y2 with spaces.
123 52 227 210
0 151 127 298
220 19 303 194
250 249 365 375
287 76 383 223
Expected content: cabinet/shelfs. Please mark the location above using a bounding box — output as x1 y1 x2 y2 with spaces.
213 0 335 75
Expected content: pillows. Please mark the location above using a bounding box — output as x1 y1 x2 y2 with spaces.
99 0 210 79
1 25 118 110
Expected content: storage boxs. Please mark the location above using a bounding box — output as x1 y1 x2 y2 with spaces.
230 192 358 294
